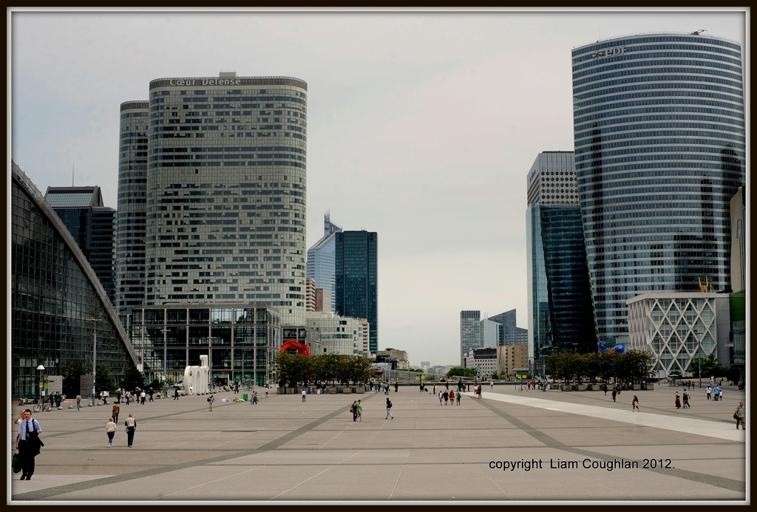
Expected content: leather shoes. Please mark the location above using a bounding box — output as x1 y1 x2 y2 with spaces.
20 472 32 480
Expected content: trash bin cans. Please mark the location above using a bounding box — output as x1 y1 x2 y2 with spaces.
243 393 248 401
352 388 356 393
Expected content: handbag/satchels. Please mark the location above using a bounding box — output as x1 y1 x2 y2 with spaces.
12 452 22 473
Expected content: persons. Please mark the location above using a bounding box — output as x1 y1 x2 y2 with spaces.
443 388 448 406
349 400 358 422
76 392 81 411
15 408 43 480
49 391 54 407
363 379 391 395
704 384 722 400
474 383 481 399
383 397 394 419
114 384 154 406
472 383 477 398
631 394 640 412
251 391 257 404
264 384 268 397
111 401 120 423
524 377 548 393
356 399 362 421
53 391 61 407
394 382 398 392
437 389 443 406
675 390 681 408
172 387 178 400
301 388 307 403
455 389 461 406
732 400 745 430
249 390 255 405
681 388 690 408
611 387 617 402
448 388 455 406
206 391 214 412
124 413 136 448
232 384 239 394
614 380 635 391
14 409 25 426
105 417 116 445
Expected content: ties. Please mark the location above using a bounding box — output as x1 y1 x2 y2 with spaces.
26 420 30 441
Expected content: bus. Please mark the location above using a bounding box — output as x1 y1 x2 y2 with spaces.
513 368 531 380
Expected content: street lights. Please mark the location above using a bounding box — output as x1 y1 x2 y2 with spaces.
87 318 102 407
159 329 171 388
694 332 705 389
36 365 45 405
653 336 661 389
